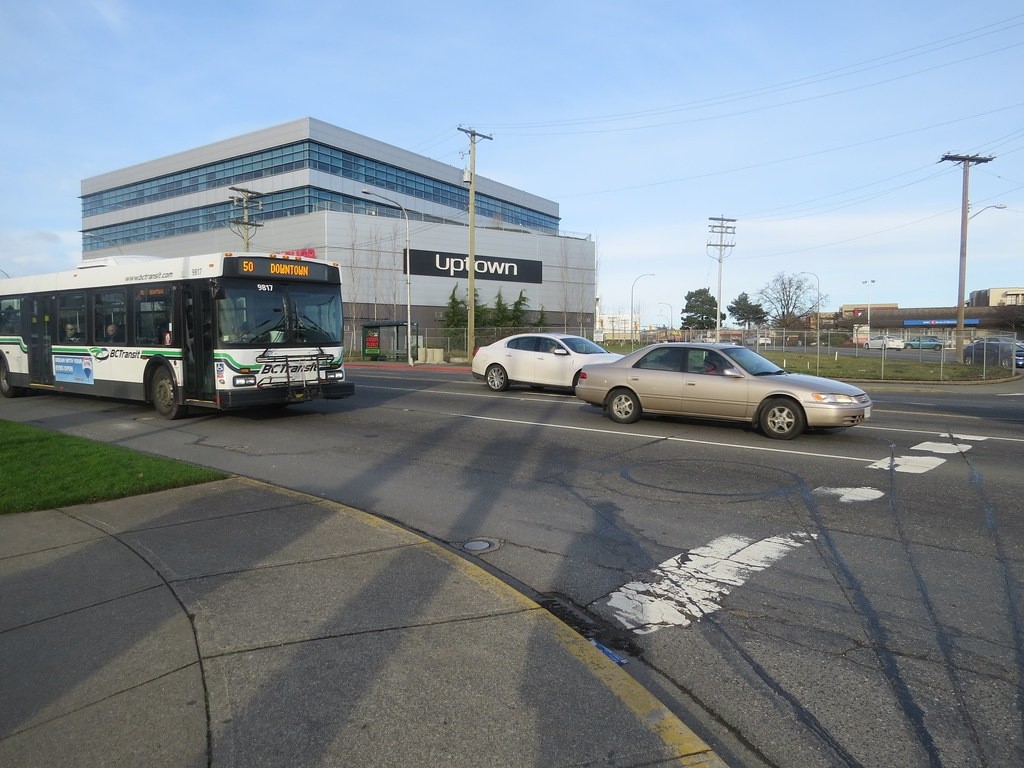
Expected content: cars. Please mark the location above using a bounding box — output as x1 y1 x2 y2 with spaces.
574 343 874 440
902 335 953 351
862 335 905 351
744 334 771 345
470 331 627 397
963 336 1024 369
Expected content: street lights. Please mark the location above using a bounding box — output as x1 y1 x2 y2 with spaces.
631 272 656 352
861 278 877 334
800 270 820 377
658 302 673 331
362 188 413 364
956 203 1008 368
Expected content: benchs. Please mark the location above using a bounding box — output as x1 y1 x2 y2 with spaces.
377 349 407 362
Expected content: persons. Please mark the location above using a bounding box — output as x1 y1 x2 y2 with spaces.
62 322 86 342
104 323 124 343
704 352 730 375
153 317 172 346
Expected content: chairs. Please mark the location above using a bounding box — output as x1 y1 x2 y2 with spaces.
547 343 556 353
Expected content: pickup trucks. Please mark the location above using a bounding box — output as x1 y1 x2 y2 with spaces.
691 333 716 344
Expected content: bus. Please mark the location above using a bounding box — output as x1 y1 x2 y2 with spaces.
0 250 356 421
